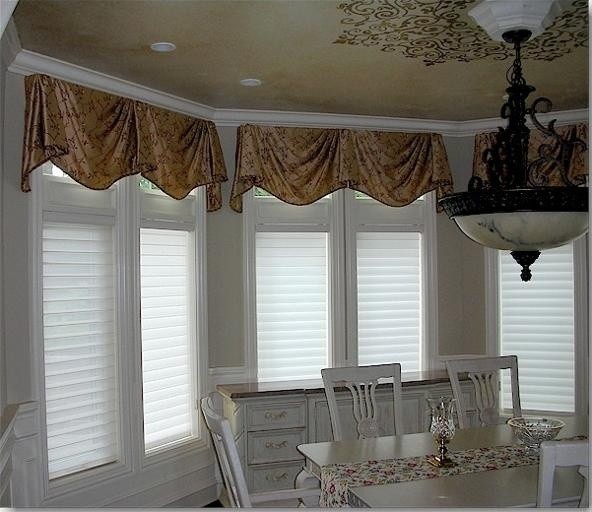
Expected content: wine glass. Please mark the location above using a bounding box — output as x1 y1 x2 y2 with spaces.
426 395 457 469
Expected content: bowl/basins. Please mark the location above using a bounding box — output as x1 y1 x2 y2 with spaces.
505 416 566 457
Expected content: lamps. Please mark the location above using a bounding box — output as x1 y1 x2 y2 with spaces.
437 1 589 283
